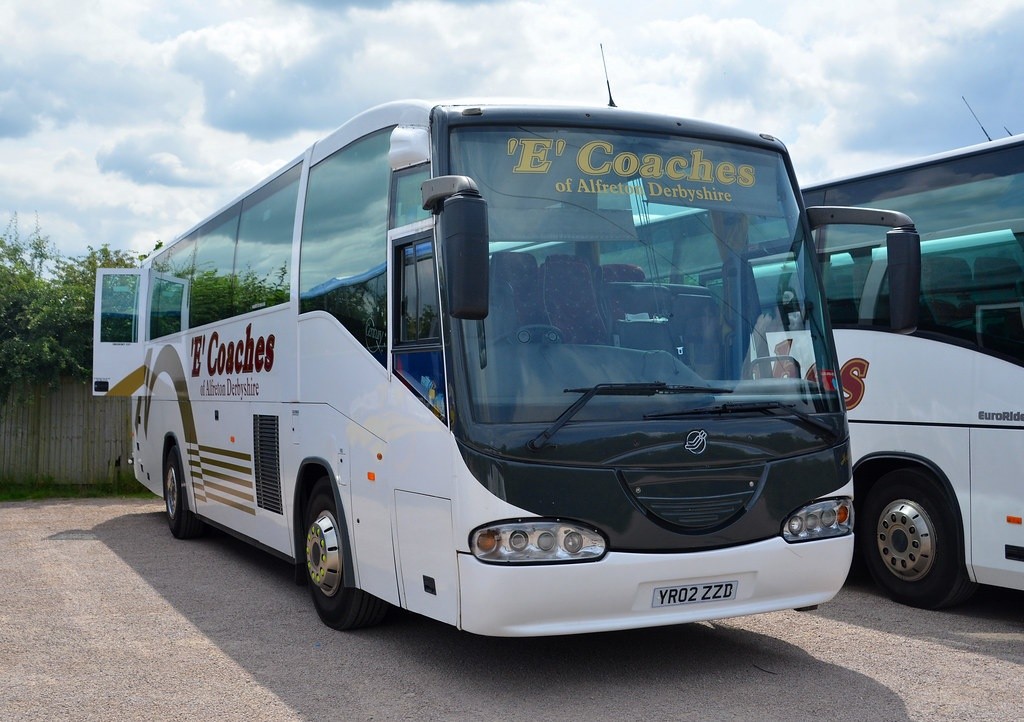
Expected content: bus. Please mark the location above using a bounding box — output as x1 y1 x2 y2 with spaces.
489 133 1024 612
93 98 923 637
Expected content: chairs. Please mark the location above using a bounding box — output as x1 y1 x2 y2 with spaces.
672 294 732 379
489 251 671 346
828 255 1024 349
